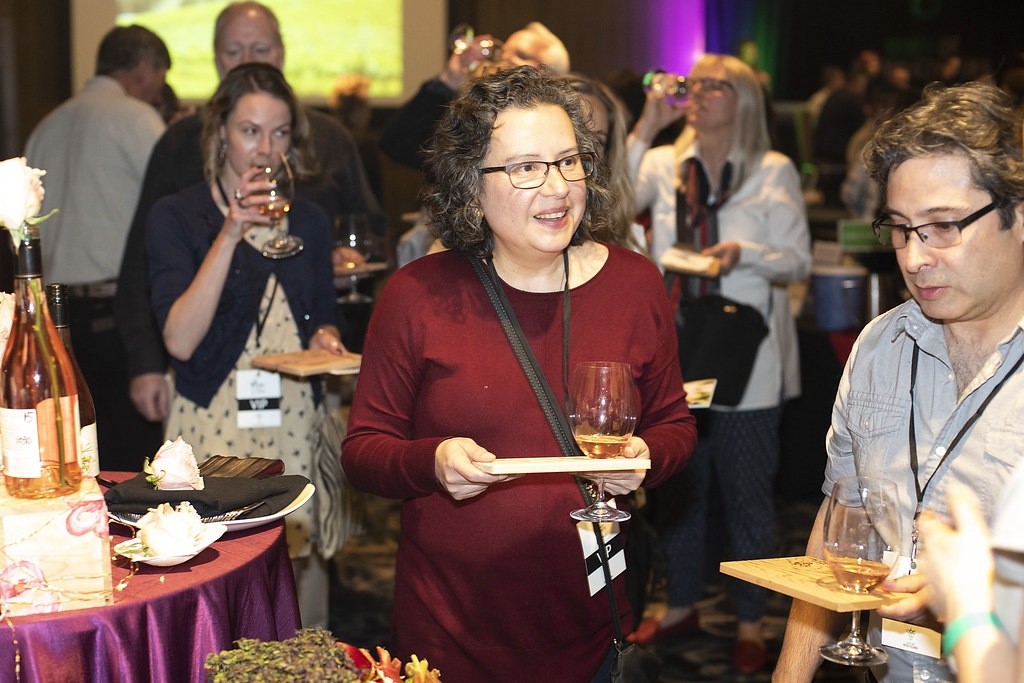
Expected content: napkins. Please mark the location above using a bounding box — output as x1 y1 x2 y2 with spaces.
103 470 310 523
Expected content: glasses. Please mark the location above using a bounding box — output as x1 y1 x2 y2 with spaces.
482 151 596 190
871 202 998 249
688 75 737 93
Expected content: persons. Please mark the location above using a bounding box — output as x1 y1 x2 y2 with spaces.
23 0 814 683
340 67 697 683
773 81 1024 683
803 45 991 213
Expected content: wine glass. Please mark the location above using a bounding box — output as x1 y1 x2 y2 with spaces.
334 212 373 304
817 476 904 667
247 148 304 260
568 361 636 522
448 22 505 72
643 67 697 108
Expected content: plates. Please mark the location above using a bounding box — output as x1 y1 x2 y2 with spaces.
104 483 315 531
114 524 228 567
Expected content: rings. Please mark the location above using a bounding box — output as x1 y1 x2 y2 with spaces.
235 190 243 199
239 198 247 208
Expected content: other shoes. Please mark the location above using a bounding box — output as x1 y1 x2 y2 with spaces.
625 606 701 643
737 628 769 677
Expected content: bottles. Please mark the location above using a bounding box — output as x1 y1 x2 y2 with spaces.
45 282 100 478
0 225 83 500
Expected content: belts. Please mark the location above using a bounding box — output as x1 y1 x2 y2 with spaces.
61 281 119 303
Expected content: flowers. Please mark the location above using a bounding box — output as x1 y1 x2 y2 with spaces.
143 436 205 491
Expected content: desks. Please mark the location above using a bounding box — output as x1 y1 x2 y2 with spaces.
0 471 302 683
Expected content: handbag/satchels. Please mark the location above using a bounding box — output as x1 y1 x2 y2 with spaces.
675 293 769 406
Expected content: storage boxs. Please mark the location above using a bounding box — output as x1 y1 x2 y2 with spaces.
0 478 114 617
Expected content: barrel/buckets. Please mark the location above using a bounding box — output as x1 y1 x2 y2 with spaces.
812 265 870 331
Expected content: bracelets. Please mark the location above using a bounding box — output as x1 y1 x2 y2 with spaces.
939 610 1007 672
